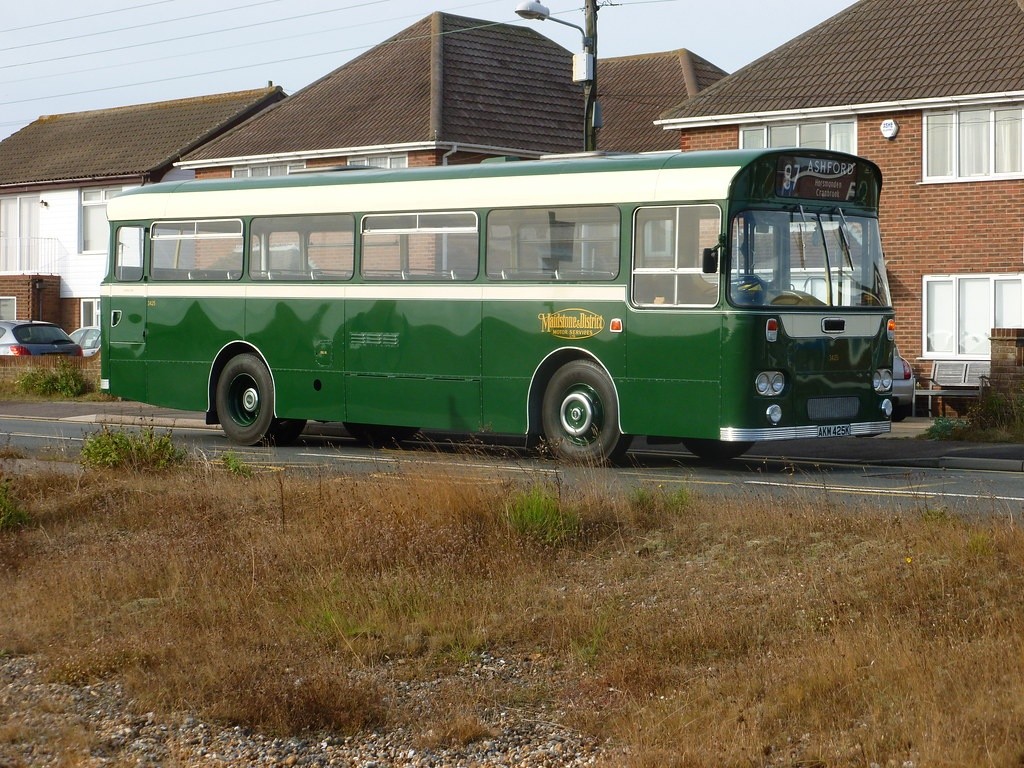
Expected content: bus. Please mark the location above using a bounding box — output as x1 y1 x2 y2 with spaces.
95 149 896 471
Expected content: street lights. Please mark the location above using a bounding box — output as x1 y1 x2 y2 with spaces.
515 0 602 156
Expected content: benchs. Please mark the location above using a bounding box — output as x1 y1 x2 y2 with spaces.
912 361 991 417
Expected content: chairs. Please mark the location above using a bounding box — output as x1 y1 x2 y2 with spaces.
187 270 615 281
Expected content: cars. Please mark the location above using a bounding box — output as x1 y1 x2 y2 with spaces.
67 326 101 357
0 320 83 358
890 345 916 423
